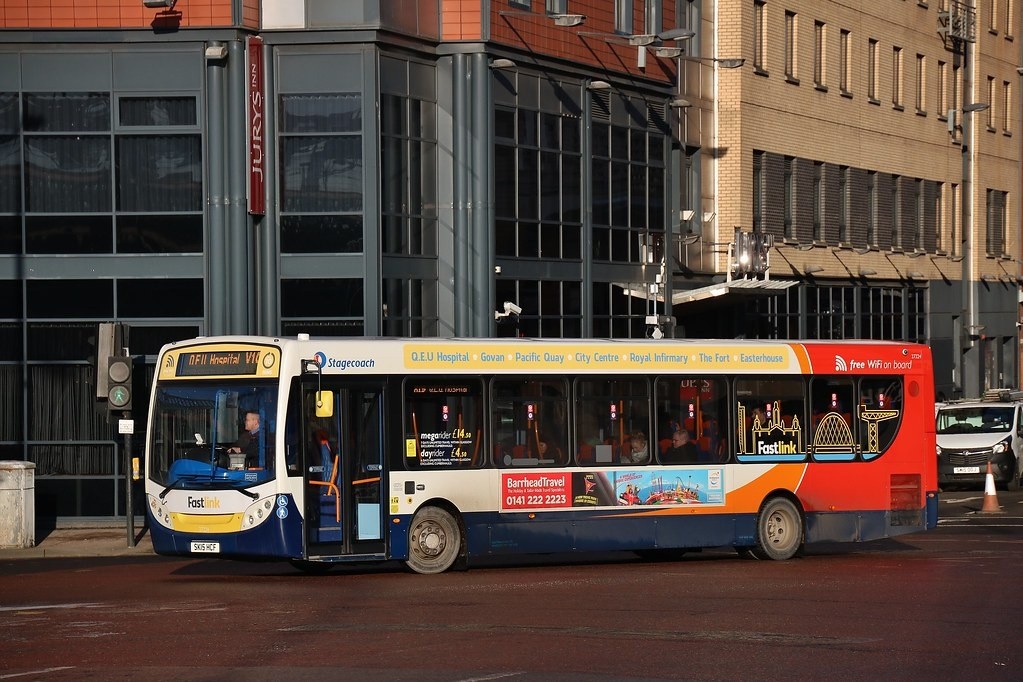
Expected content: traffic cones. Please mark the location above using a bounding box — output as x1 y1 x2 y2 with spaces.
978 462 1003 512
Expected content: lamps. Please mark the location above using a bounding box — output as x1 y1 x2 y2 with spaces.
854 245 878 257
884 247 906 256
669 100 693 108
795 241 829 249
489 59 516 68
204 46 227 58
832 243 855 253
906 249 929 258
981 274 996 280
585 80 612 90
143 0 175 8
773 237 801 249
858 268 877 276
922 248 948 259
1000 274 1012 278
804 266 825 274
906 271 924 279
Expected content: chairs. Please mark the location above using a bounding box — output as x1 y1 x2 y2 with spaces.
512 444 527 459
702 415 716 437
660 439 673 461
579 444 596 463
697 438 715 461
684 417 695 439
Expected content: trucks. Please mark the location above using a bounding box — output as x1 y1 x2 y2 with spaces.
935 389 1023 493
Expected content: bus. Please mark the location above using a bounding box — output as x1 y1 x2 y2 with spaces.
143 333 939 574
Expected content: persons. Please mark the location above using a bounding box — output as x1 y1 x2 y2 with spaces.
532 435 560 463
227 412 260 468
746 401 778 452
665 429 698 461
604 438 631 464
631 433 648 462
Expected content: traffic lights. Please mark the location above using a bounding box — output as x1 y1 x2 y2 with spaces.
107 357 132 411
